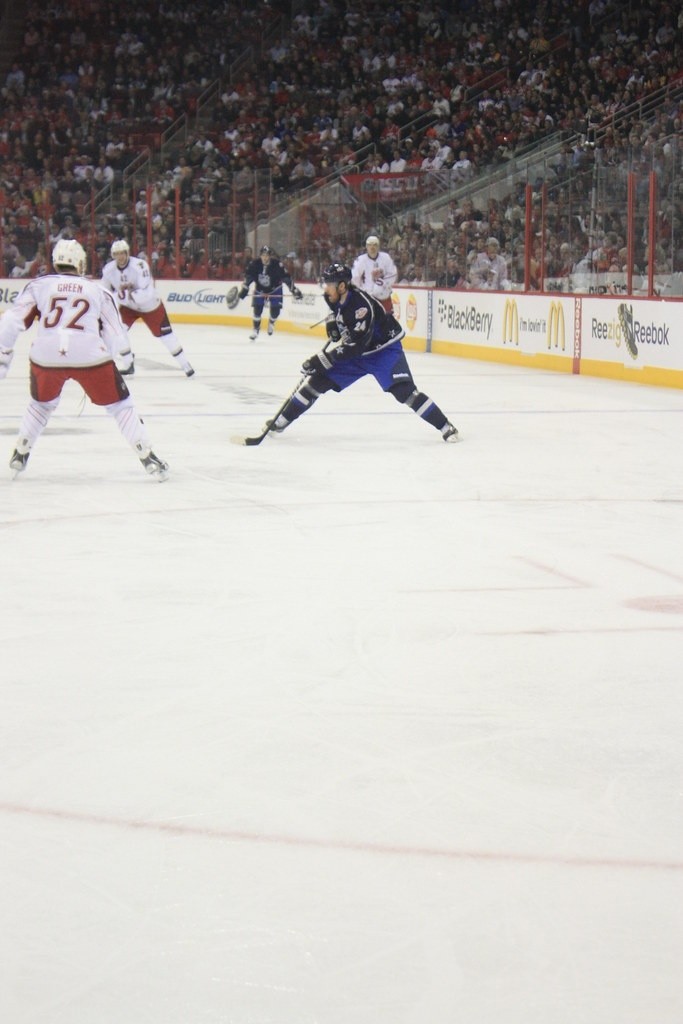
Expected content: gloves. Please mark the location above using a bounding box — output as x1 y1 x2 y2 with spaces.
292 287 303 300
326 321 342 343
238 288 248 299
0 349 13 378
300 351 336 376
375 277 388 290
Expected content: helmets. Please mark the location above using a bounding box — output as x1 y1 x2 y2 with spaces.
365 236 379 249
110 240 130 261
260 244 272 256
485 237 499 249
51 238 86 276
318 263 352 291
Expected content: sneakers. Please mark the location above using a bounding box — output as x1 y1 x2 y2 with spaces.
185 363 195 381
261 414 290 438
439 418 458 442
119 354 135 378
268 320 275 335
249 328 260 342
134 442 167 483
8 437 31 480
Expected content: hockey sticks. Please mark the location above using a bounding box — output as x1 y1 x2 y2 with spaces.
246 294 295 297
229 367 315 445
310 315 334 330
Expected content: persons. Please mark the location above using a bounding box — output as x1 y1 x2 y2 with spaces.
103 241 196 379
238 245 304 341
352 237 397 315
0 239 170 482
262 262 460 443
470 238 510 291
0 0 683 288
